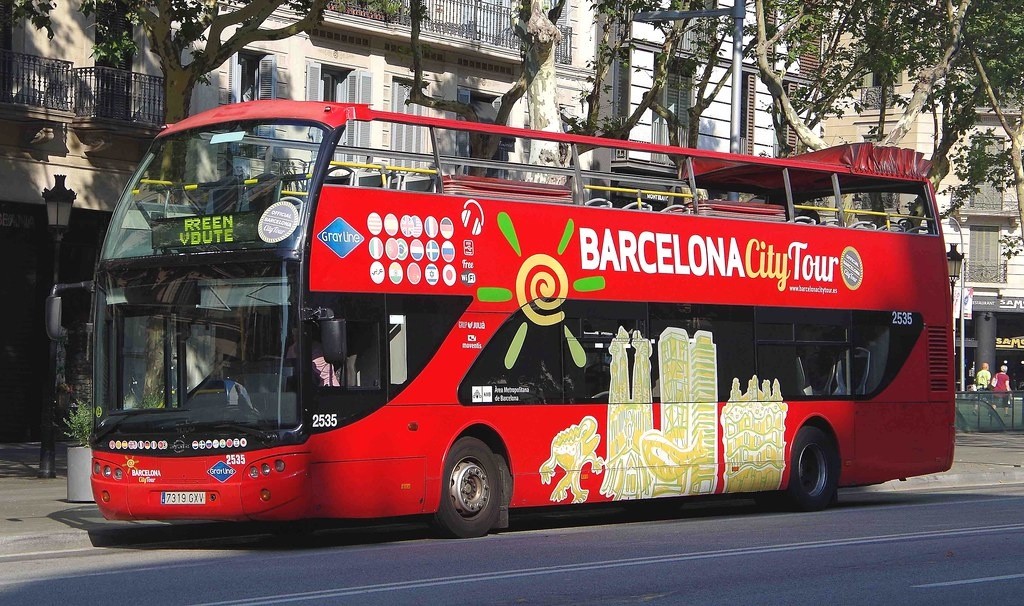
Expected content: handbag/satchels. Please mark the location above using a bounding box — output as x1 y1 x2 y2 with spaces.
988 373 998 389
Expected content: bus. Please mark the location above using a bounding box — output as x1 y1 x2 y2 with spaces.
44 97 959 538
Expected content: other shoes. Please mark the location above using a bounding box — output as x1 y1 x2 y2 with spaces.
988 413 991 416
973 410 978 416
1004 414 1011 417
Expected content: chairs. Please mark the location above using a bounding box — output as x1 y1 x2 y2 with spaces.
795 346 870 396
787 217 934 236
204 162 689 212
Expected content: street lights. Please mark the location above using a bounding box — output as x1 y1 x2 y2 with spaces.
946 241 966 322
36 172 77 477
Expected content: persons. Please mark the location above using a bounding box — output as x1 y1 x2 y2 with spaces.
287 322 340 387
798 195 866 228
972 363 1019 417
